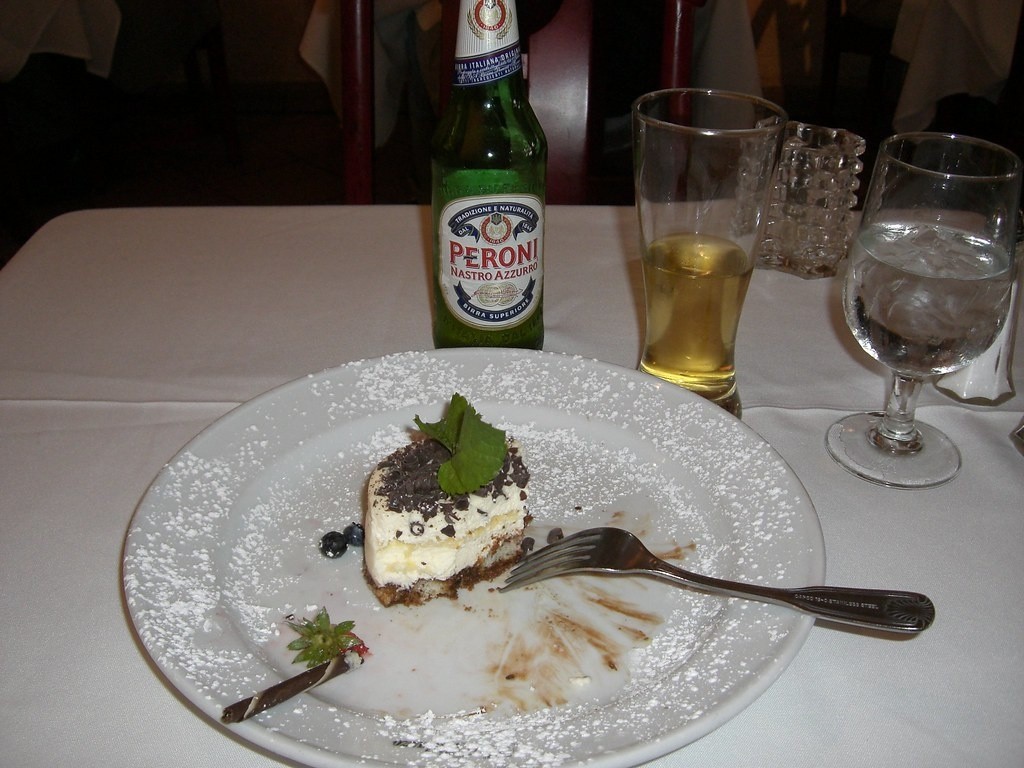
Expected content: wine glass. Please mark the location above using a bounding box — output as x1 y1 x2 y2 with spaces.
826 133 1021 489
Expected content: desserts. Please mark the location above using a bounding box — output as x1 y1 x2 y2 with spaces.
362 433 532 607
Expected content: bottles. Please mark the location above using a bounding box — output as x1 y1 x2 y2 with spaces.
933 210 1017 404
429 0 547 349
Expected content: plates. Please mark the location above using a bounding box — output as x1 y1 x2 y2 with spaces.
121 347 826 767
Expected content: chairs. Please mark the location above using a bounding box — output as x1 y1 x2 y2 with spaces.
341 0 693 204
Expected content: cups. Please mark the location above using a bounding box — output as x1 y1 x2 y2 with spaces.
632 87 789 421
752 120 865 278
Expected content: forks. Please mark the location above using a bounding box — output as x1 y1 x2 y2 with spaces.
500 527 934 635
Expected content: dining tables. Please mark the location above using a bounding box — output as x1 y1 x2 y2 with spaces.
0 205 1024 768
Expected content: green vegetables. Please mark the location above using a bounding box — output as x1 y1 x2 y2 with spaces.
413 392 509 495
